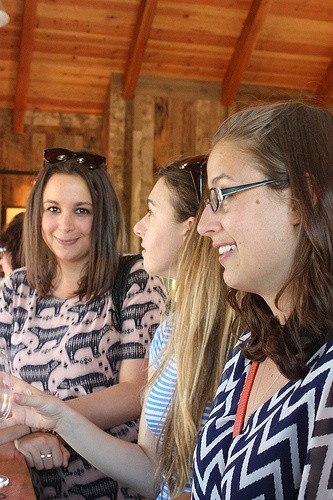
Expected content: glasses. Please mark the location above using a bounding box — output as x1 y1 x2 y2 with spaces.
208 182 278 211
44 146 107 175
168 156 206 202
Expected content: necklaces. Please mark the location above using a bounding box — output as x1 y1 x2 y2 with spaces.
242 356 283 430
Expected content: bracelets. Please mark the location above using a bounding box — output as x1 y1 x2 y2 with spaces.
29 425 32 435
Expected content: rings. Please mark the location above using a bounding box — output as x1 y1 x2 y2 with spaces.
40 454 53 458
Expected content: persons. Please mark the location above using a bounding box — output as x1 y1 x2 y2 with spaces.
0 148 171 500
0 155 246 500
169 101 333 500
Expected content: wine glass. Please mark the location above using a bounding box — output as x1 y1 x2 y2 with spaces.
0 349 11 487
